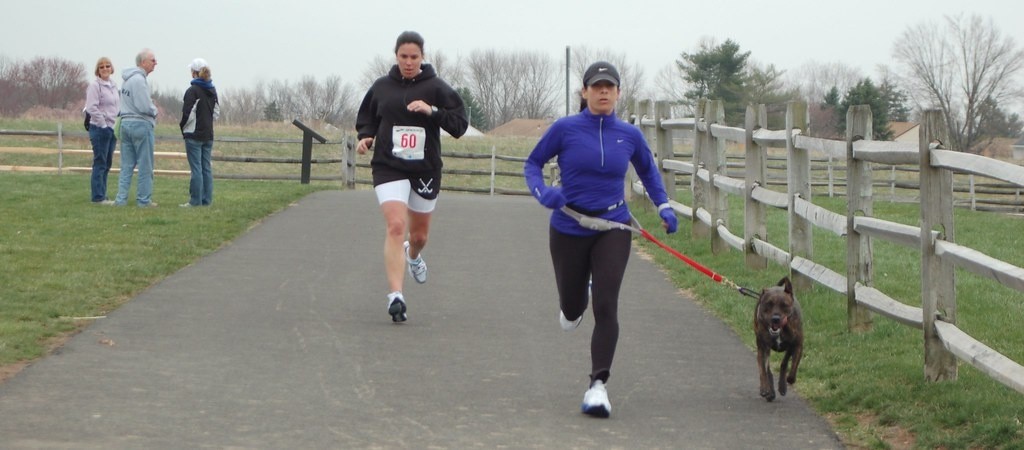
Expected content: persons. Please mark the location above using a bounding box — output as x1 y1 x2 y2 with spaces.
355 30 469 323
523 60 679 418
82 56 121 205
178 58 220 207
113 49 158 208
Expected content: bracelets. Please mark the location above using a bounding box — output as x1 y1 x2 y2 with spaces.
657 202 675 216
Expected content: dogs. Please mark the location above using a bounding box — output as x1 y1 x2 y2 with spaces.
753 275 804 402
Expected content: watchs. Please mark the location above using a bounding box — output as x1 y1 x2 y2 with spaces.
430 104 439 117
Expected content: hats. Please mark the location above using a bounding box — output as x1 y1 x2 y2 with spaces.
186 58 209 72
582 61 620 87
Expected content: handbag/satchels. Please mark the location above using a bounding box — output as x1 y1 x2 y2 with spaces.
83 111 92 132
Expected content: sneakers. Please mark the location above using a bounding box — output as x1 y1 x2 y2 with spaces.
402 240 428 284
559 279 592 332
581 383 612 418
387 291 408 322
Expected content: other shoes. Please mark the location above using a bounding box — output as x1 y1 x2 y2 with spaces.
91 198 114 205
147 202 158 208
178 202 193 208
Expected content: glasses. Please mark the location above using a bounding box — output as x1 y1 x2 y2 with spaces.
98 64 112 69
147 58 156 63
